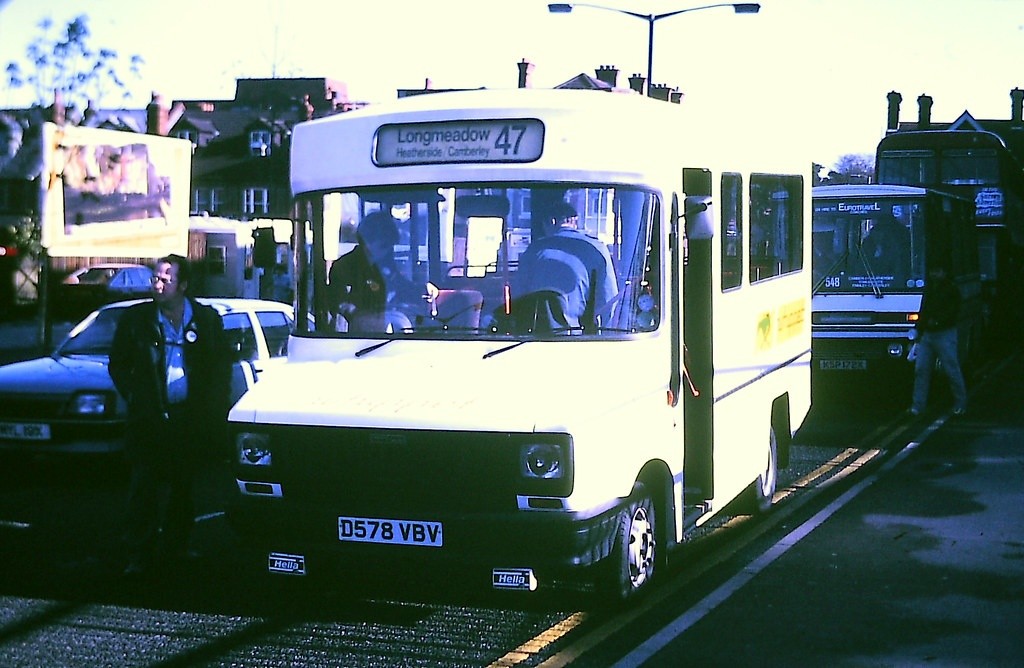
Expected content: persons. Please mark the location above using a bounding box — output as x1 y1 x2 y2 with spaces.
108 253 225 580
328 212 401 334
904 262 968 416
518 202 621 335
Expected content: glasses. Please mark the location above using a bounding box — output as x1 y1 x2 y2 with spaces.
151 276 178 284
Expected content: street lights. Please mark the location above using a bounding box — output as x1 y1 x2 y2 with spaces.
548 0 761 100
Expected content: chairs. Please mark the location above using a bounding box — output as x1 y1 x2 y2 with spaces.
398 265 626 331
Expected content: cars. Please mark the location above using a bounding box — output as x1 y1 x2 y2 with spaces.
47 260 153 300
1 298 298 477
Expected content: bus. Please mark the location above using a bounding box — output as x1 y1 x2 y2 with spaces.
764 183 974 377
872 126 1021 335
222 93 817 595
